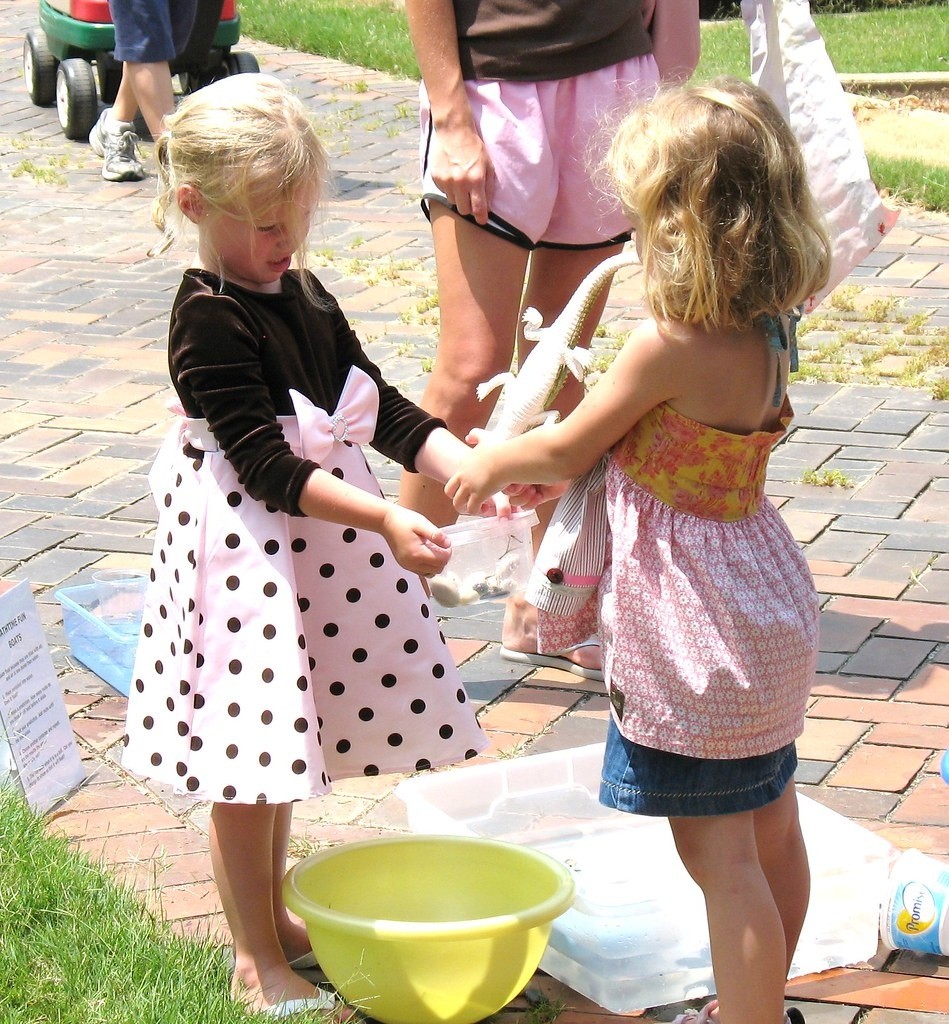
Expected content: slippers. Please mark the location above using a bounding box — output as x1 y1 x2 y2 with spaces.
231 949 335 1023
499 635 605 680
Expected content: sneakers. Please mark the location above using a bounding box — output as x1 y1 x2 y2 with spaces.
89 107 143 180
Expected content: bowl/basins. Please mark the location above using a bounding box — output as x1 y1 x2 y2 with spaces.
94 568 156 632
415 511 543 609
880 847 949 959
277 835 574 1022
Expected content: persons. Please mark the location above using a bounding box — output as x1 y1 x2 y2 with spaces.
139 71 527 1017
80 0 206 182
437 75 842 1024
394 1 705 689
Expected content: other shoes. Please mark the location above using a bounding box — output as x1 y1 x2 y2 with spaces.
669 1000 716 1024
